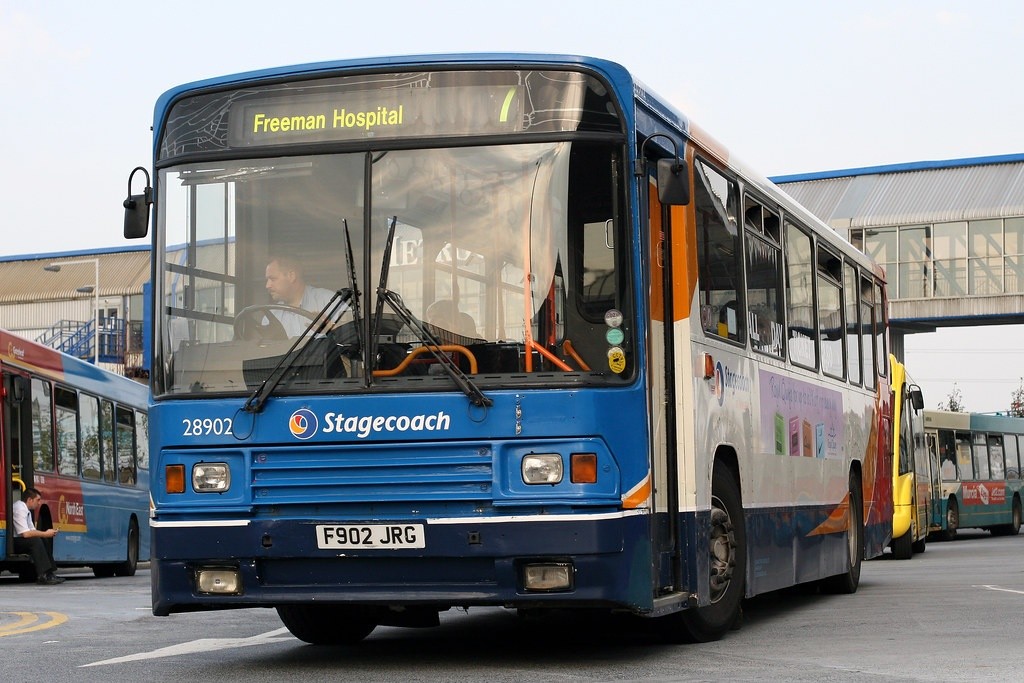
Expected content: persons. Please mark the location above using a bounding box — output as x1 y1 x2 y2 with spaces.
260 253 364 378
410 299 490 376
12 487 66 586
719 300 739 342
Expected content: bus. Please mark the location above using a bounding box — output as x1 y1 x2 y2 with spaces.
921 410 1024 542
123 54 893 652
0 329 151 582
886 354 932 564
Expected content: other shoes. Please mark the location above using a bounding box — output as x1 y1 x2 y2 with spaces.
38 574 65 585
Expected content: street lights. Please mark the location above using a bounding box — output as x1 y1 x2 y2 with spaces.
44 257 99 367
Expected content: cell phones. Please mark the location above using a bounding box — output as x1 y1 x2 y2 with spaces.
55 526 61 532
